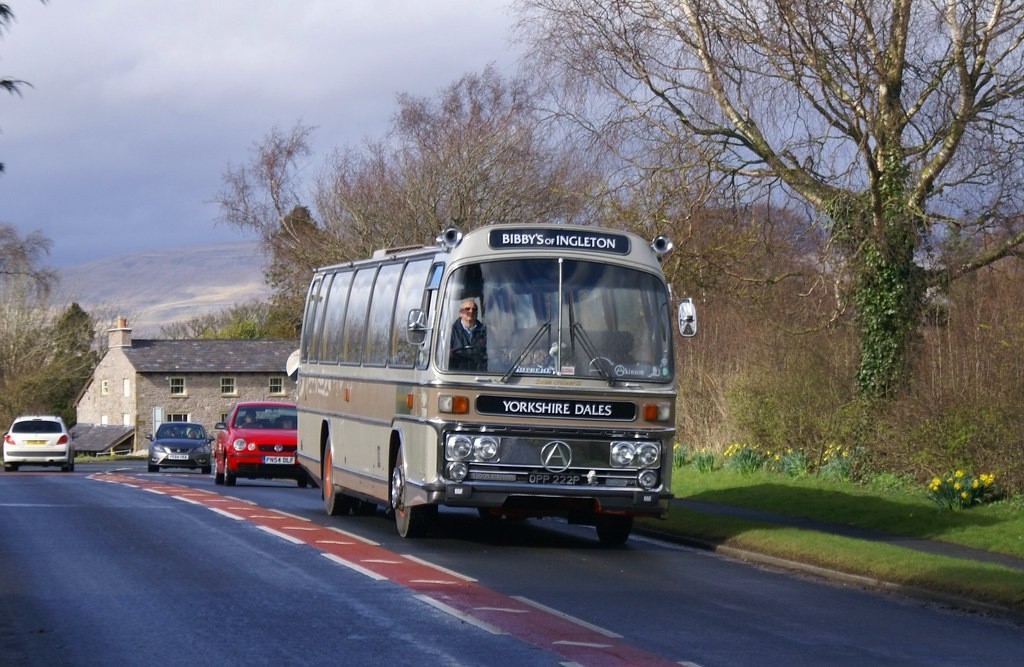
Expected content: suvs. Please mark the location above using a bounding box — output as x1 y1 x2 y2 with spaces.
3 414 79 473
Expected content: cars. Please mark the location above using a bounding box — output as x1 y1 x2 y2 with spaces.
145 421 215 474
215 400 298 487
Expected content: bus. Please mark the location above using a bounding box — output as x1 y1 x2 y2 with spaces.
295 223 698 546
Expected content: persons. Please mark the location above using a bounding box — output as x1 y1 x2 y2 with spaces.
238 409 265 428
280 417 292 429
450 299 498 371
166 426 199 438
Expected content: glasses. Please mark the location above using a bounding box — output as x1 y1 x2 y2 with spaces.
462 308 477 311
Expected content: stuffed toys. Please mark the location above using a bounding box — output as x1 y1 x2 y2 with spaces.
543 341 567 375
